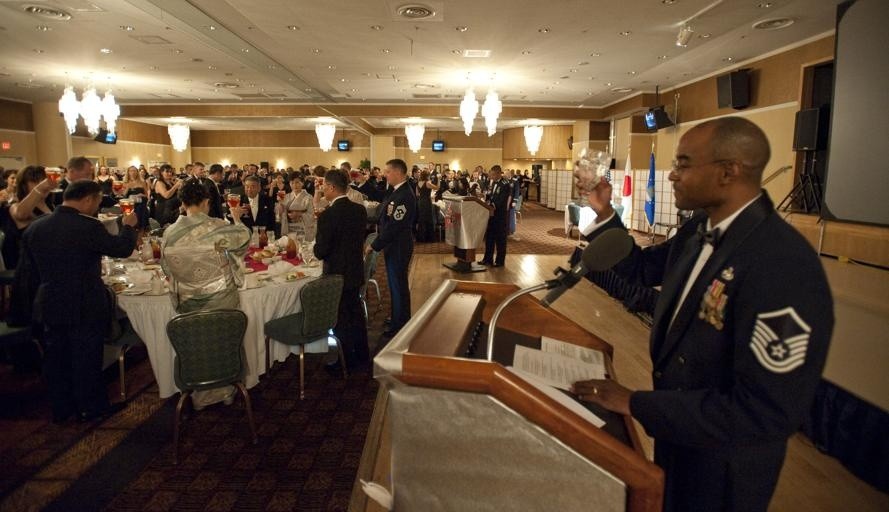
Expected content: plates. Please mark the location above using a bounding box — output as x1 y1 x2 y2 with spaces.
113 288 170 296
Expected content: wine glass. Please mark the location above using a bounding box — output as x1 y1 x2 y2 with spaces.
101 259 114 279
45 168 64 193
112 181 123 198
228 194 246 220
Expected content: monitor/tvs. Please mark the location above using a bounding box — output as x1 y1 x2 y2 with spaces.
95 129 117 144
338 141 349 151
432 141 444 151
644 111 673 130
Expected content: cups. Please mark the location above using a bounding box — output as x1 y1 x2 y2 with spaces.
262 258 272 265
120 199 133 216
272 256 284 262
246 275 256 289
152 280 164 293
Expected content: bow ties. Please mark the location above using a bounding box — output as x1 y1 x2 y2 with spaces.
494 181 499 184
695 222 720 250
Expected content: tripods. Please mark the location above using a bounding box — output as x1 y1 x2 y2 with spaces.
776 152 820 212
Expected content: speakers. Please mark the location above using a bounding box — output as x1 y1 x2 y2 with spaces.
792 108 829 152
717 72 749 109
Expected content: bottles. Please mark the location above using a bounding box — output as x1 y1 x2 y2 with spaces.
139 236 161 263
251 225 274 248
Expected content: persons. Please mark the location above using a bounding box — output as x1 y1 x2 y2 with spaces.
0 165 68 269
571 117 834 510
363 158 416 339
313 170 370 375
410 161 532 268
160 179 251 410
93 162 328 242
7 179 139 416
342 162 386 202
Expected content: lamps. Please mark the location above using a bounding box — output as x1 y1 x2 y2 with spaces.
313 85 544 158
676 25 695 49
59 84 192 154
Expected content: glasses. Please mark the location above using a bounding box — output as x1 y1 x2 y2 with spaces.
671 159 733 176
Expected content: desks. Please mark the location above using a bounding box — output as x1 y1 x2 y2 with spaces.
562 200 627 249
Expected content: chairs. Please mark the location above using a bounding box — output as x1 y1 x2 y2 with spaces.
165 307 258 462
0 182 527 467
361 233 382 319
263 274 345 397
1 182 526 260
1 260 150 401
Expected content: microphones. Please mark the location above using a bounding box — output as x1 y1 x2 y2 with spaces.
486 228 634 360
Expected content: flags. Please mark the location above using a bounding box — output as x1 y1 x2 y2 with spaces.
644 152 656 229
620 151 632 218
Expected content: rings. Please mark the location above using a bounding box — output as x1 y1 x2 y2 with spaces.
594 388 598 396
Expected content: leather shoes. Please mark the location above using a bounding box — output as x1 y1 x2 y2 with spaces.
477 260 504 268
324 358 370 375
383 316 397 339
